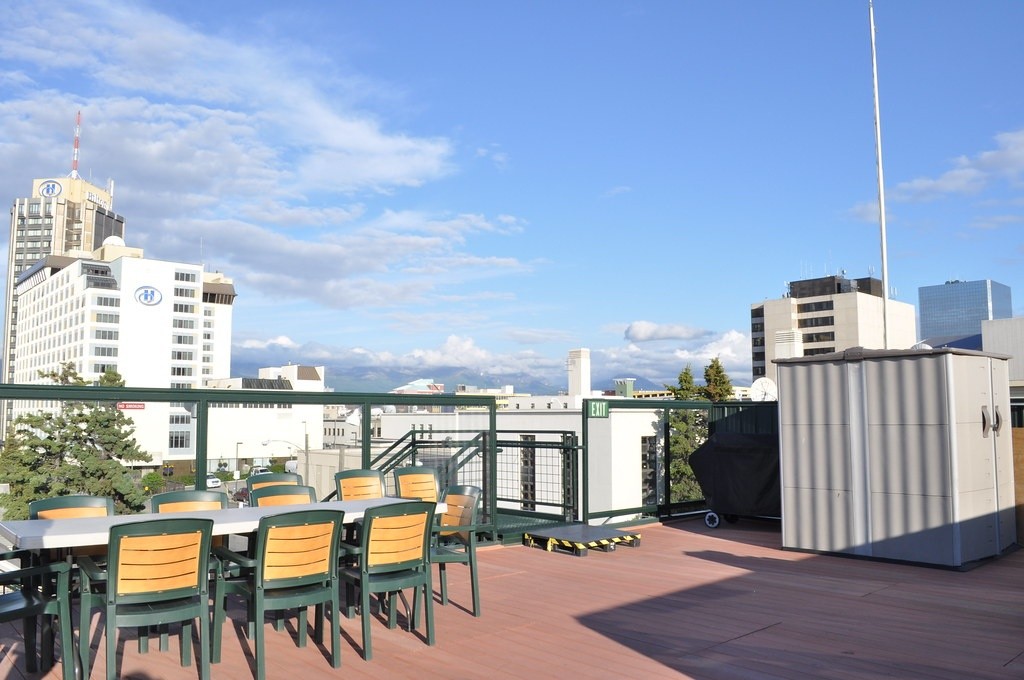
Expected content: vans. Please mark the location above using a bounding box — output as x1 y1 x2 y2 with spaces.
234 486 249 503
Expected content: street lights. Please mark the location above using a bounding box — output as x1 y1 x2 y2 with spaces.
237 441 243 492
263 420 309 486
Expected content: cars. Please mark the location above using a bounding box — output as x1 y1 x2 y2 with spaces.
205 473 222 490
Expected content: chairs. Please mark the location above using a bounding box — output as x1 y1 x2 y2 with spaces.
29 495 115 593
393 467 437 501
411 486 481 629
246 473 302 505
211 510 345 680
335 470 385 500
152 491 228 513
252 486 317 507
77 518 214 680
0 550 75 680
340 502 436 660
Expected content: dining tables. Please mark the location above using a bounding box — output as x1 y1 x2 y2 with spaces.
0 498 447 671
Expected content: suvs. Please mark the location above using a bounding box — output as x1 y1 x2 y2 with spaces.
250 467 274 477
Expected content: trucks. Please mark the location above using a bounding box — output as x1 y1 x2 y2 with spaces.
284 460 297 474
